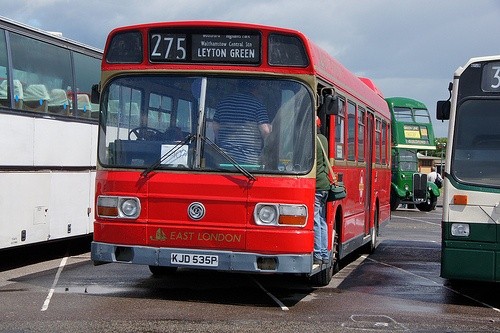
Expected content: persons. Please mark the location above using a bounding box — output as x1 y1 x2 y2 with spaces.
213 80 270 165
312 116 329 265
427 167 443 209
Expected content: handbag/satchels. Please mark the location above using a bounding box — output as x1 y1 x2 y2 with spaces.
327 181 346 201
435 174 444 189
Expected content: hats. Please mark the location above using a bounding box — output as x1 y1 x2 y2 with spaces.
309 116 322 128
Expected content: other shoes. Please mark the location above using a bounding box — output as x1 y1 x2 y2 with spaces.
313 256 329 264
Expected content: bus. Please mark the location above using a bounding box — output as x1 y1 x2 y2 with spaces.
437 54 500 290
385 96 441 212
0 17 172 252
90 20 392 288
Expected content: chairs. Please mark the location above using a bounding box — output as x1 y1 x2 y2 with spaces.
0 80 173 131
